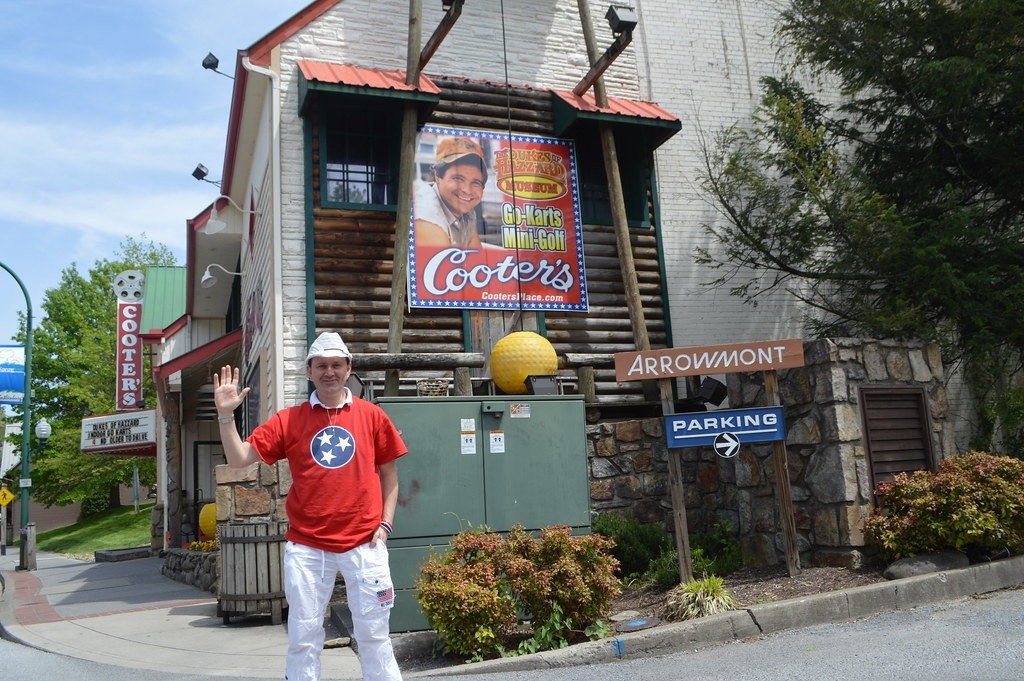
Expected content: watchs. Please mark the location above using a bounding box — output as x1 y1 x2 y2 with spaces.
218 415 234 423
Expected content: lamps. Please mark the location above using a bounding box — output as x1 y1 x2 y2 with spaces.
201 50 219 69
192 162 209 179
205 194 261 235
135 400 146 408
603 3 640 33
200 264 247 287
84 409 94 416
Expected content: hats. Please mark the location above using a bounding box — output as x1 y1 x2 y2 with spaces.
305 331 352 382
436 137 487 183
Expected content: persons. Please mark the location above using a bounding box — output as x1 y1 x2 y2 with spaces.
214 332 408 681
414 138 488 249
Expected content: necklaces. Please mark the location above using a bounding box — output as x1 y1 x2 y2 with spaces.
325 403 340 439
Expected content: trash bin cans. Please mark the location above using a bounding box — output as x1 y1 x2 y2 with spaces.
219 521 290 626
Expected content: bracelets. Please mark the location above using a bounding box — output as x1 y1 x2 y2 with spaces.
380 519 393 536
